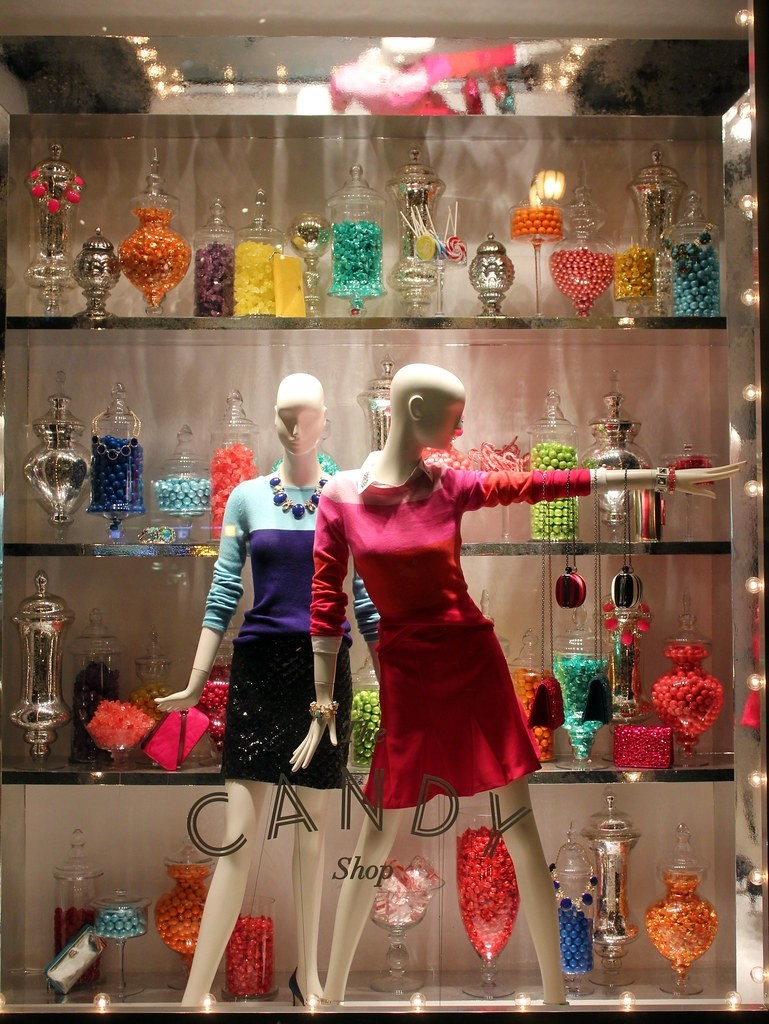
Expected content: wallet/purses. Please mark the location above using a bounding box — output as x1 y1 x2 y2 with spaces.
141 706 209 773
270 252 307 319
44 925 108 995
611 724 676 769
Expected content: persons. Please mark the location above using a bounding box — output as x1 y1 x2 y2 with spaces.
327 36 562 116
288 363 747 1006
153 374 381 1007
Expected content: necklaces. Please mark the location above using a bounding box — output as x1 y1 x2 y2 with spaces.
29 159 85 214
602 599 651 646
269 476 328 520
91 409 142 460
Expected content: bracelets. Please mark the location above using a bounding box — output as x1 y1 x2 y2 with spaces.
656 465 676 494
307 700 340 718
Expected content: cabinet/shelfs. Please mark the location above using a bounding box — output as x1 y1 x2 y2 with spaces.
0 113 742 1017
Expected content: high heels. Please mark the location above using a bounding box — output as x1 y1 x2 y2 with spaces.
288 967 304 1006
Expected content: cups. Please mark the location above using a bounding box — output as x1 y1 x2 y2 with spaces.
220 895 279 1004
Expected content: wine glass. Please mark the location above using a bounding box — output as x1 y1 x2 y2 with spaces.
370 878 446 994
453 806 520 996
